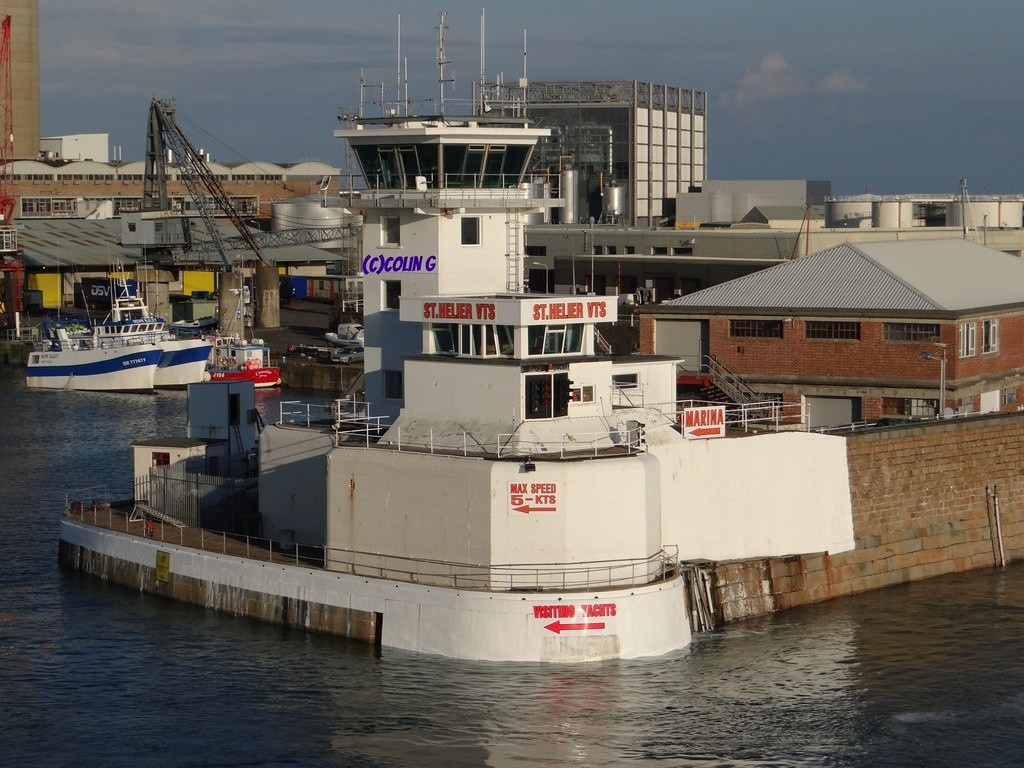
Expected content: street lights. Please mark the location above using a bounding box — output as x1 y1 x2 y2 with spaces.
931 343 947 418
534 261 548 294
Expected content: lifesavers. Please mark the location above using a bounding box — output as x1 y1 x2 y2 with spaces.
221 357 228 366
228 357 237 367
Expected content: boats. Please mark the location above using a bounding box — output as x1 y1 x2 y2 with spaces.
325 322 364 347
208 337 282 387
90 278 214 391
24 318 162 394
166 315 218 335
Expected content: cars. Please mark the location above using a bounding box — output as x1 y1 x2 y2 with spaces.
331 345 364 365
